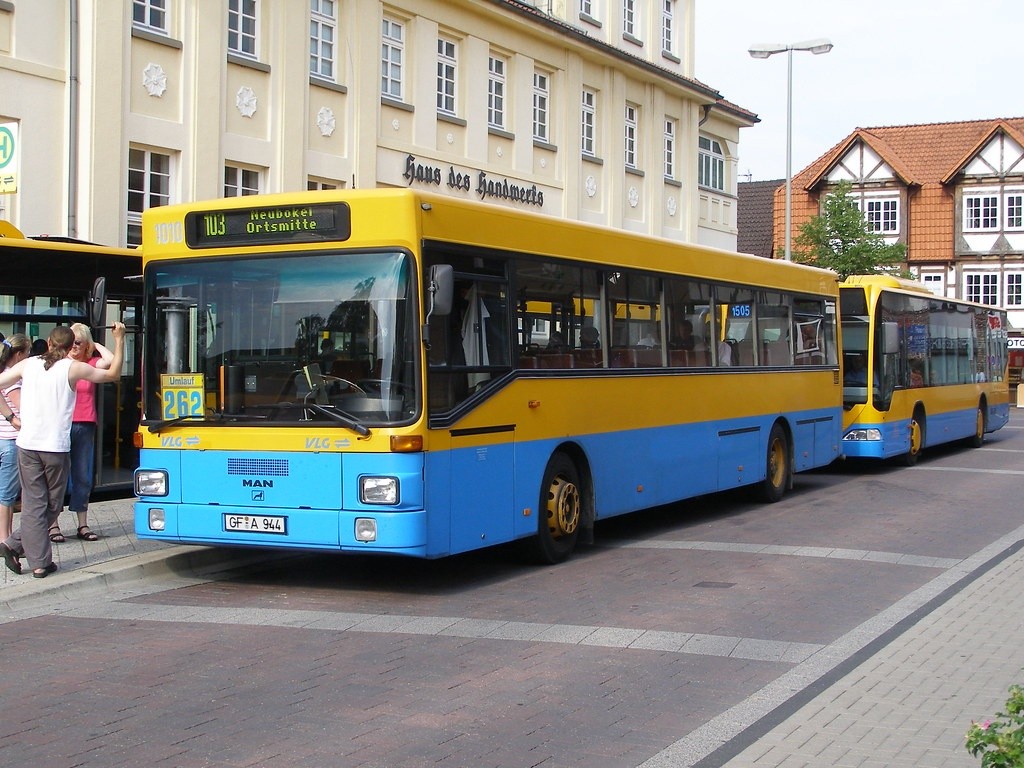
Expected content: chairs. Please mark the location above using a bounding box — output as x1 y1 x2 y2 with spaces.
228 325 825 419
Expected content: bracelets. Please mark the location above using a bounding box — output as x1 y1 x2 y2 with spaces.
5 413 15 421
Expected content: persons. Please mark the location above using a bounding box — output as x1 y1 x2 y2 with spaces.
579 327 600 349
0 319 126 578
542 331 567 354
693 321 732 366
0 333 31 560
28 338 48 360
319 339 335 358
0 331 6 342
670 320 700 351
47 321 114 542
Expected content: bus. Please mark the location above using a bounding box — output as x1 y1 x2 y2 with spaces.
835 276 1008 464
0 219 143 510
135 190 845 566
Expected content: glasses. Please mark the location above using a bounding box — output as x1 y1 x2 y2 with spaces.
74 340 82 345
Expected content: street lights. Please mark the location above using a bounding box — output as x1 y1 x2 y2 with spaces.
748 39 832 261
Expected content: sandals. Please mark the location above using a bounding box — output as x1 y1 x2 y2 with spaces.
48 525 65 542
77 525 98 540
33 562 57 578
1 542 22 574
